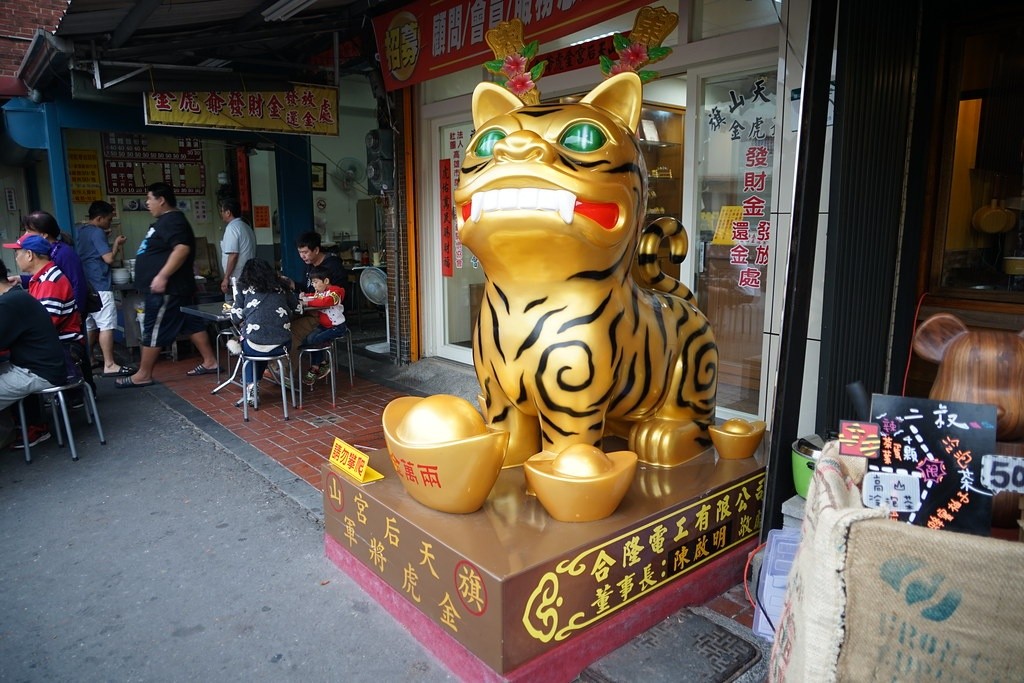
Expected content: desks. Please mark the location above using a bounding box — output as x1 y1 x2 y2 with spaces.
347 265 388 315
180 301 328 407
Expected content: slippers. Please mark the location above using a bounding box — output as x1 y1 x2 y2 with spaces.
186 363 223 376
102 366 137 378
91 360 103 369
113 374 153 388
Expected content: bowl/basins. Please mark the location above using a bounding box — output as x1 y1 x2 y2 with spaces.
123 259 136 282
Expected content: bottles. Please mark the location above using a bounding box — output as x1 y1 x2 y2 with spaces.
354 241 362 263
362 242 370 267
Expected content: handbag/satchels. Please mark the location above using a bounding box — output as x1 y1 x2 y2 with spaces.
87 280 103 314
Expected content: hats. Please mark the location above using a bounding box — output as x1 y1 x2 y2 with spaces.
2 231 51 257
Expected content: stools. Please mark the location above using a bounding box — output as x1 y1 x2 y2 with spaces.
19 376 106 465
216 327 355 423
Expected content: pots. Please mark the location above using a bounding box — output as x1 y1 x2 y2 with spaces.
111 267 130 285
792 431 838 501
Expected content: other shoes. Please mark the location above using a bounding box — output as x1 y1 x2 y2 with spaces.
70 398 97 408
44 393 69 407
247 383 260 407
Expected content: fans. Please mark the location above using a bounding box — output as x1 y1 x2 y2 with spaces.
360 267 391 354
335 157 366 191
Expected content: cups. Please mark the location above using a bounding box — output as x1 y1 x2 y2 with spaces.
372 252 380 267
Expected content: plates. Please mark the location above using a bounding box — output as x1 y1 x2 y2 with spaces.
127 199 138 211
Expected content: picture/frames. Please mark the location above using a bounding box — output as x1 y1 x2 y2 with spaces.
311 161 327 191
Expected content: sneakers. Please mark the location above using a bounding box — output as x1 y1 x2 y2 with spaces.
10 423 52 449
10 413 41 426
306 368 317 385
318 363 332 380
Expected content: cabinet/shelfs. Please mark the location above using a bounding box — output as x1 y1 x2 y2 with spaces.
632 98 686 229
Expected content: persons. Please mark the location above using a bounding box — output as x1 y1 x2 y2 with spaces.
264 231 350 380
231 257 297 407
77 200 137 377
298 265 346 386
0 259 67 412
10 233 84 449
7 212 98 409
114 182 223 389
220 200 257 358
